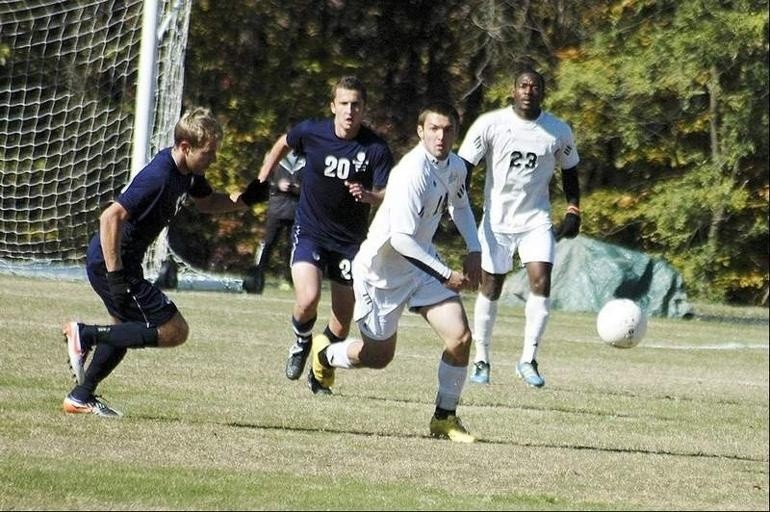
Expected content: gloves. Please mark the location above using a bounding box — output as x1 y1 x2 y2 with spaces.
105 270 137 313
447 212 461 237
240 178 271 206
557 205 582 241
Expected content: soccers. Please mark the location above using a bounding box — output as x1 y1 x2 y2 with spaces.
597 298 647 349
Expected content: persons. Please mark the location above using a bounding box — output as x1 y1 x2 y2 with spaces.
62 107 270 419
455 69 581 389
255 125 308 290
312 101 482 445
253 77 396 398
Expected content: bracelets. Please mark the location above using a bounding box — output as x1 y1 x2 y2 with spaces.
565 205 581 216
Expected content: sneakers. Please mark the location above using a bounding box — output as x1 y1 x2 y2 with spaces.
285 335 312 379
307 369 333 397
62 396 125 420
469 360 491 383
312 332 335 387
516 360 545 388
428 414 476 445
63 321 88 386
